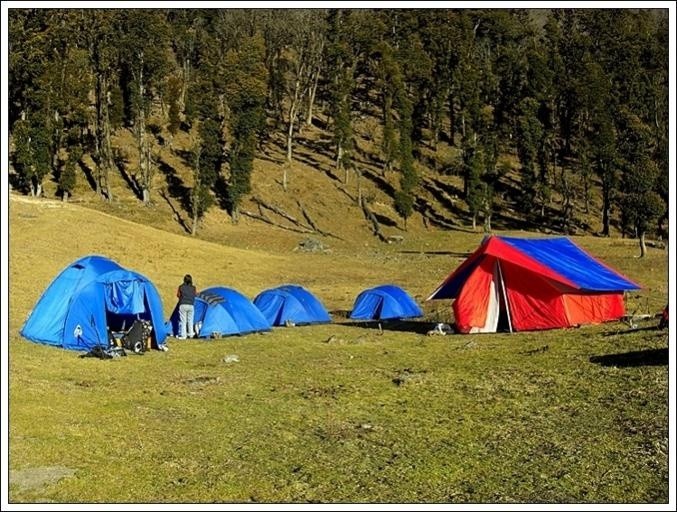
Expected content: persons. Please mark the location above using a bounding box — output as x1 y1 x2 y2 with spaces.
176 273 197 342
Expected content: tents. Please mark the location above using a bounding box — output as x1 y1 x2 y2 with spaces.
167 287 274 341
346 283 425 322
16 253 169 354
253 284 332 328
425 233 648 334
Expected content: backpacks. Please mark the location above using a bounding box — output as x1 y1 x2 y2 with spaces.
121 319 152 352
